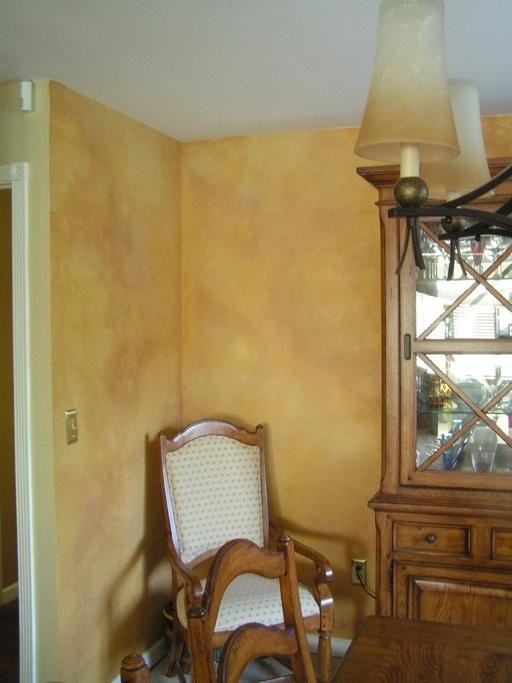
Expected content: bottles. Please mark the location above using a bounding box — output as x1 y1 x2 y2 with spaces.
490 365 509 415
471 426 497 472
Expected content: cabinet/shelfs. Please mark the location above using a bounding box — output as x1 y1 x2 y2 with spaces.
374 508 511 632
356 156 512 501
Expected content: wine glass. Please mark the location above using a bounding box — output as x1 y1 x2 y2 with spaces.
420 222 507 279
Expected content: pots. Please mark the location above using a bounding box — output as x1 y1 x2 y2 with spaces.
450 383 483 411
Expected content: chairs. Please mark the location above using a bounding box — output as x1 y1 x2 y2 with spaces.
159 419 333 682
120 652 150 682
188 531 317 683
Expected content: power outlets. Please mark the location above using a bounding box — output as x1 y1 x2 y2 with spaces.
352 559 367 585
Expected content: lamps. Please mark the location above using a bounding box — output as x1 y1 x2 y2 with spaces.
354 1 511 281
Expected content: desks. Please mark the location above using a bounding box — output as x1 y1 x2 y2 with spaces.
331 615 512 683
